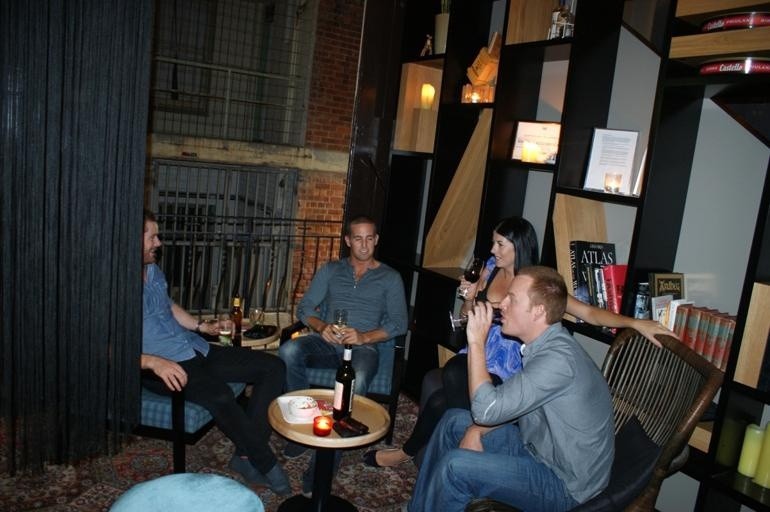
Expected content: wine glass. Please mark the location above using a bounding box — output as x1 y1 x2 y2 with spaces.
449 302 504 332
456 257 484 300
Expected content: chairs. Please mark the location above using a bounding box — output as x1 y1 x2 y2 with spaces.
280 322 406 443
108 382 246 473
464 325 725 512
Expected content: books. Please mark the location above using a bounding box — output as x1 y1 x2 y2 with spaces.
567 239 735 371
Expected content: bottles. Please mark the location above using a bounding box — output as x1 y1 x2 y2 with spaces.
549 0 575 40
231 293 242 335
332 344 356 421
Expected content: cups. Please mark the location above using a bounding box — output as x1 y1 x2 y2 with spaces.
313 416 334 437
521 140 539 164
333 310 349 340
248 306 265 330
217 312 234 348
602 169 624 195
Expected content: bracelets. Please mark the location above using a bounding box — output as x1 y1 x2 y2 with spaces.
194 319 206 334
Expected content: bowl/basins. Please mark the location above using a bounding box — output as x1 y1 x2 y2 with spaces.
288 397 318 418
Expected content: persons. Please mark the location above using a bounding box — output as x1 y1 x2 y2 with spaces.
142 214 293 498
404 265 615 512
362 213 681 469
277 211 410 497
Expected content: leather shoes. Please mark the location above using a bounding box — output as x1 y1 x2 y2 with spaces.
363 448 406 467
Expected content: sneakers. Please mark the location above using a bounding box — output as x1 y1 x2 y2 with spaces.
228 455 290 495
283 441 308 459
302 480 314 497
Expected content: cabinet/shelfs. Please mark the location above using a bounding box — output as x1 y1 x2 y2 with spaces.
380 1 770 512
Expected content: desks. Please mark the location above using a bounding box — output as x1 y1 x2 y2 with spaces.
213 319 281 347
267 389 391 512
